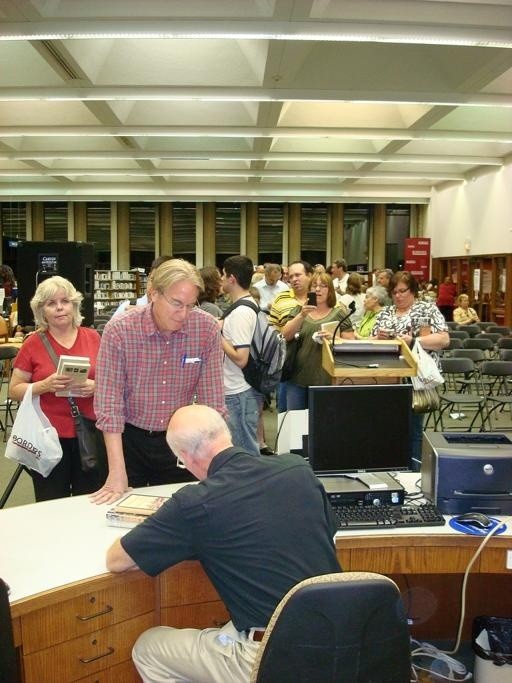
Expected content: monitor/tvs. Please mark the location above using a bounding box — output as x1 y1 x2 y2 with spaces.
308 385 412 489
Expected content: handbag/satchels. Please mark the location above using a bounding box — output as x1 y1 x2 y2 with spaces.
75 414 107 472
279 335 303 383
4 383 64 479
411 387 440 412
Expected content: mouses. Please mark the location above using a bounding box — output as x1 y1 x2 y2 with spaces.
456 512 493 529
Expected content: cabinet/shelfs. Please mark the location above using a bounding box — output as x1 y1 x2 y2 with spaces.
93 268 139 323
133 270 151 298
430 249 511 329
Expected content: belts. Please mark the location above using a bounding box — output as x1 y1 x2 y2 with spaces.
244 629 266 643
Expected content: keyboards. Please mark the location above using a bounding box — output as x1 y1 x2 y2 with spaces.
333 505 445 530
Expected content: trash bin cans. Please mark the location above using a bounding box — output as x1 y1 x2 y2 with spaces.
472 615 512 683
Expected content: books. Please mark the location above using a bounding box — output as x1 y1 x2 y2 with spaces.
53 362 89 399
91 268 146 316
56 353 91 376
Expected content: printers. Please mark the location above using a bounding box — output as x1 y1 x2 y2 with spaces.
422 431 512 516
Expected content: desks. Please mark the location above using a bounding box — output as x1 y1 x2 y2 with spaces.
0 471 512 683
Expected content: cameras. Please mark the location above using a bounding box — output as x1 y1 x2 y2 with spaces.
384 329 396 338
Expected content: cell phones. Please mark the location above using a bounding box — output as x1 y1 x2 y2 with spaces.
307 292 316 306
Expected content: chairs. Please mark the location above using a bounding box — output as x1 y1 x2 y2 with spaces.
0 285 144 448
245 567 419 683
387 312 512 438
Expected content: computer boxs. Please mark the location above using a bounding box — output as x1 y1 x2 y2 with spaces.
313 473 405 508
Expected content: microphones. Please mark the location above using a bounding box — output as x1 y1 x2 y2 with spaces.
332 300 356 358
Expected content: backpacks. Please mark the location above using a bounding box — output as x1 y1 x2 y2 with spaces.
411 336 445 391
219 299 287 393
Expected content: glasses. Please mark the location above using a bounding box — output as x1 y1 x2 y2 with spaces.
176 455 187 469
160 290 201 312
392 285 410 295
311 283 328 287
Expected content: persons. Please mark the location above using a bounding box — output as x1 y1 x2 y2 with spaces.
85 254 227 505
105 404 342 681
8 276 108 499
